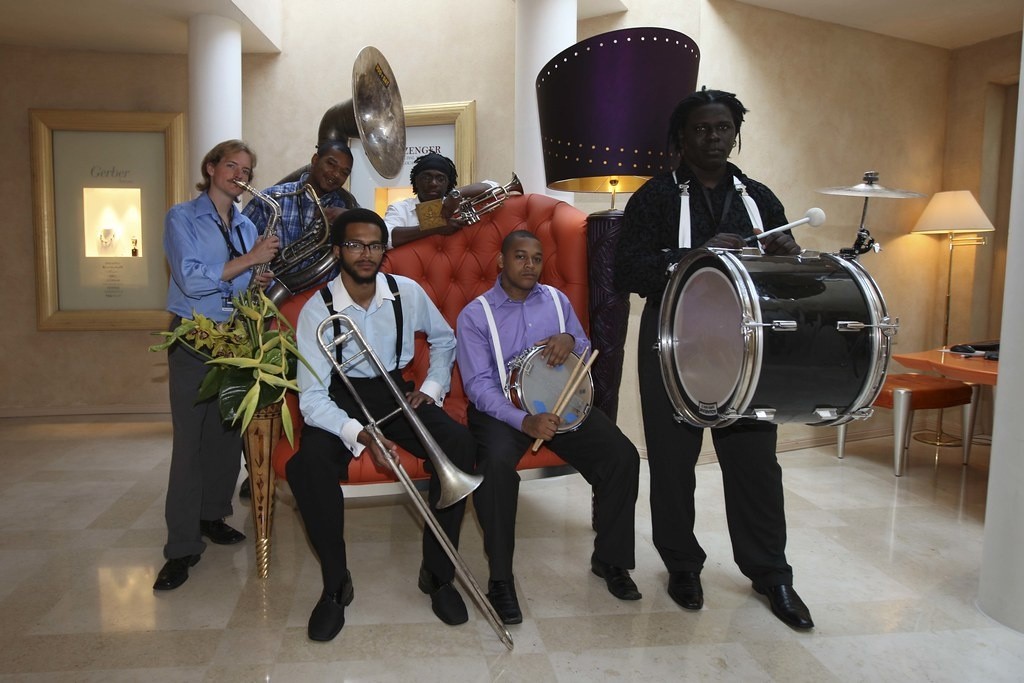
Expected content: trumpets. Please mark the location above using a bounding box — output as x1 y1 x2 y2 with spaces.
441 170 525 225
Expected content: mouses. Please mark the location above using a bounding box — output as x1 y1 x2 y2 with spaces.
950 344 975 353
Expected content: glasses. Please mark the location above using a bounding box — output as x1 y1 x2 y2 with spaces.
338 240 388 254
415 172 450 186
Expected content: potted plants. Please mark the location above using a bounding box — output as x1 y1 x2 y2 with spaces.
150 285 328 580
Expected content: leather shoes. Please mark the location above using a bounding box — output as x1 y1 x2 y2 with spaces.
308 569 354 641
668 569 703 610
487 574 522 624
752 580 814 629
418 559 468 625
200 518 246 545
591 550 642 601
152 553 201 590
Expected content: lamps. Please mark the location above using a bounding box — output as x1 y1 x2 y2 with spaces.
909 190 995 449
538 27 701 534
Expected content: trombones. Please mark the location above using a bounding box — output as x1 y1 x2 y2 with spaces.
315 313 516 652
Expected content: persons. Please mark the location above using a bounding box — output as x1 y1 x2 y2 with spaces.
457 228 643 626
283 209 469 645
617 90 815 631
241 140 363 281
155 139 279 592
383 149 501 251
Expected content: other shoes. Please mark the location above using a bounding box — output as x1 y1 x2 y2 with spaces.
239 475 251 498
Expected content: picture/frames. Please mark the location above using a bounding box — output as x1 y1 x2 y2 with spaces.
28 106 191 332
335 101 474 246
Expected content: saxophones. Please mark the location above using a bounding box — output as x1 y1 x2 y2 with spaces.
231 174 294 308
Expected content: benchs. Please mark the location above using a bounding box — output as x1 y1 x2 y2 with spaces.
225 194 640 578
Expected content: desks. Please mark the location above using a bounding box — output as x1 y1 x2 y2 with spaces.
891 340 997 465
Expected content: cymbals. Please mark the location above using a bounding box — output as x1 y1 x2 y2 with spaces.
814 184 927 201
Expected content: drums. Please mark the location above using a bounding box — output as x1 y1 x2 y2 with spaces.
657 250 890 430
505 343 595 434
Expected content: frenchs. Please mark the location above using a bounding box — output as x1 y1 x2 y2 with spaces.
269 46 406 293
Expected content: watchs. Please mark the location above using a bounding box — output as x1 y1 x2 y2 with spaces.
449 188 462 202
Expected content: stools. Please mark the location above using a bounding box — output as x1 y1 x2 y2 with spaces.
836 373 976 474
